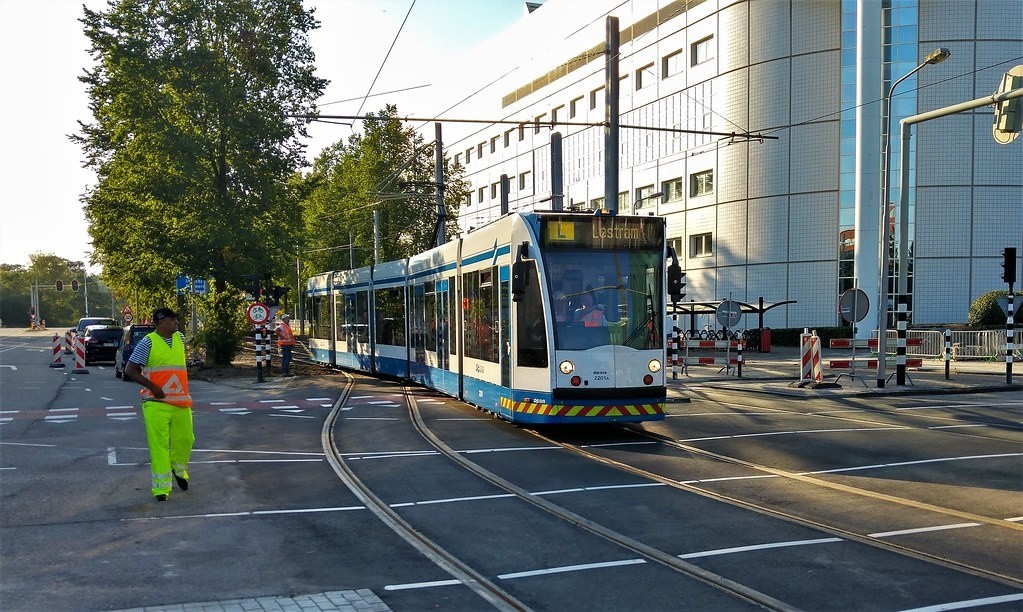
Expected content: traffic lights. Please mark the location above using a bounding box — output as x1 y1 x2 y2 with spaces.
31 308 35 315
1000 247 1016 282
242 273 259 300
56 280 64 293
275 285 291 298
671 273 687 302
71 279 79 293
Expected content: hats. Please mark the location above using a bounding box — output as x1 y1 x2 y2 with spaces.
282 314 290 319
153 307 181 323
553 290 566 300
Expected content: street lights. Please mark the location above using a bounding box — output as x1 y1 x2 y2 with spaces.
877 47 951 388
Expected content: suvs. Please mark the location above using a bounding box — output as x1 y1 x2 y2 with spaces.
70 317 115 338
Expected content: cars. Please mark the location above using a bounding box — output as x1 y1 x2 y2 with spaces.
113 324 155 381
84 324 124 366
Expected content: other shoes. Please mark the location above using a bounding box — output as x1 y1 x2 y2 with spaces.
154 494 168 501
172 469 189 491
282 371 294 377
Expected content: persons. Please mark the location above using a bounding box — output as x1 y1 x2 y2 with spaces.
553 291 567 322
479 315 493 356
273 314 296 378
430 315 448 362
574 293 611 327
518 286 546 345
125 306 195 502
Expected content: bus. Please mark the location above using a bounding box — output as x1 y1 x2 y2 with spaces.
306 180 681 426
